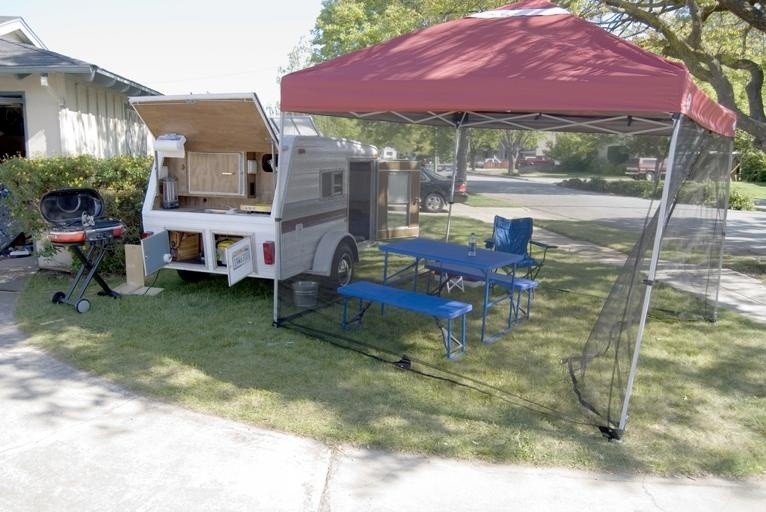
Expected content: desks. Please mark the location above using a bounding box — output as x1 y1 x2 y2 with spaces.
50 229 125 314
378 237 524 344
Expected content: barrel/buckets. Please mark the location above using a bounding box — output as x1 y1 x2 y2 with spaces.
291 281 319 308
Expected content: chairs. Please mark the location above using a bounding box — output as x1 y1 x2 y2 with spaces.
483 214 558 301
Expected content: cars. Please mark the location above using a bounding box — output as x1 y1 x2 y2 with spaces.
419 165 467 212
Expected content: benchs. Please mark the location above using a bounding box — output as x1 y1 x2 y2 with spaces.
336 261 538 359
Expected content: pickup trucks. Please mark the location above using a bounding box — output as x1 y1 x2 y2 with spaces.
518 155 555 166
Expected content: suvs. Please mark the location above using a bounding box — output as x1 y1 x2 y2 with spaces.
624 157 666 181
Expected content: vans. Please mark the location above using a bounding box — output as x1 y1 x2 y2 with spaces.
128 87 416 303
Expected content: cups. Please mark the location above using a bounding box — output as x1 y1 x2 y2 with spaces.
158 176 180 208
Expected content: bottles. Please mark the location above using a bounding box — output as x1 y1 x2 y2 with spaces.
467 232 477 257
